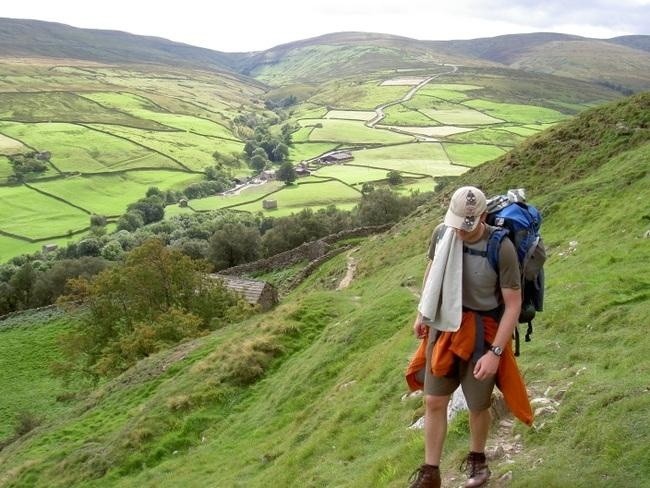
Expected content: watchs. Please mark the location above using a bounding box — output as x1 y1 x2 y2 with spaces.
490 345 503 358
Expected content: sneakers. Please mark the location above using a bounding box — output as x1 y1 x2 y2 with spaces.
409 464 442 488
458 451 493 488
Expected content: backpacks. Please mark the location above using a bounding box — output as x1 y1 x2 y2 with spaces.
430 192 549 326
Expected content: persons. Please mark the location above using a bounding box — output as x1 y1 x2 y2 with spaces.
404 186 537 486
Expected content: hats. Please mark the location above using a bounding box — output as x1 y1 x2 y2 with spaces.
444 184 489 232
